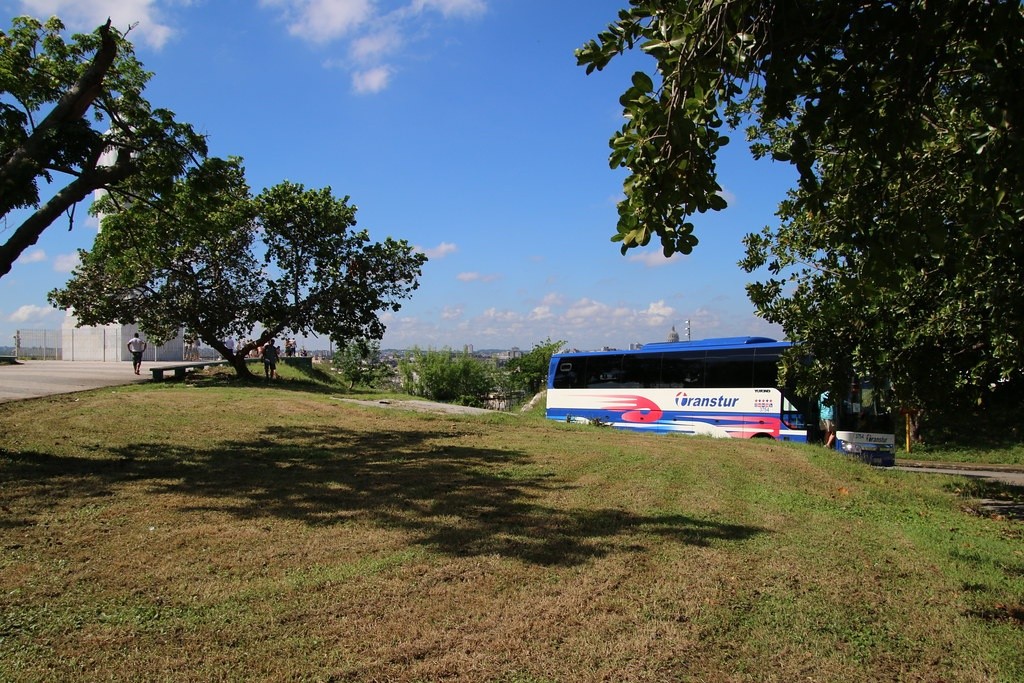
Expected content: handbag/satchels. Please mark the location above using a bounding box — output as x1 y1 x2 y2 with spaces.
270 356 278 364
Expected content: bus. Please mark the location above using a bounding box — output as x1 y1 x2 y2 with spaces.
543 336 897 468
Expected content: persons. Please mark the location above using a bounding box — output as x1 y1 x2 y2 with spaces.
237 339 264 358
225 335 234 349
276 338 307 357
127 333 146 375
262 339 279 382
818 385 837 448
11 330 22 356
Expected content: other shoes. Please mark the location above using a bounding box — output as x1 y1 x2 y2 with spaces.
824 444 831 449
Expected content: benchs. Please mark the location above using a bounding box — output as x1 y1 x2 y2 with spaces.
150 360 229 382
